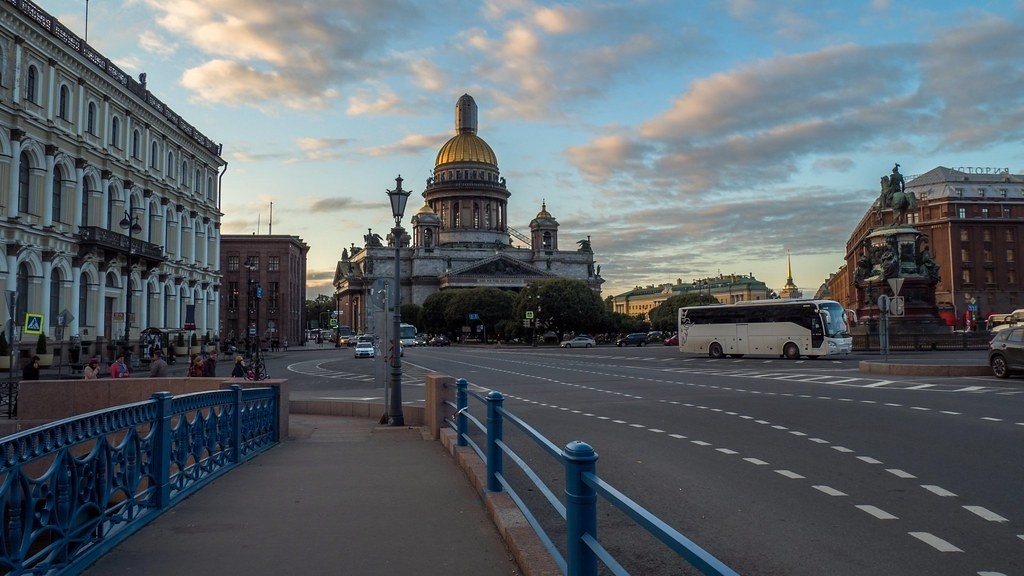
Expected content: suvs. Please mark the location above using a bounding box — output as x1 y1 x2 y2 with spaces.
616 333 648 348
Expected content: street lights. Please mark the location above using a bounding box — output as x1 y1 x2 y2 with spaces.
334 282 345 347
243 255 255 360
525 282 542 346
302 294 329 344
119 212 143 371
383 174 413 426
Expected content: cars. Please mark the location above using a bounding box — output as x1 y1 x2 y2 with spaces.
413 332 450 348
304 326 405 361
560 337 596 348
988 324 1023 380
664 335 678 347
504 329 559 345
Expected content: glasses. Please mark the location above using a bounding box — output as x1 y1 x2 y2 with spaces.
197 360 201 362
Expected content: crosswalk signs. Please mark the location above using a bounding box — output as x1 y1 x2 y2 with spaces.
24 313 42 334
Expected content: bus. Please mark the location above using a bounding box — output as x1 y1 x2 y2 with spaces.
985 313 1013 338
677 300 858 364
398 323 418 346
1003 309 1024 336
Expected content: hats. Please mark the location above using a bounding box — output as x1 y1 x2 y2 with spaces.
91 358 99 364
116 354 125 359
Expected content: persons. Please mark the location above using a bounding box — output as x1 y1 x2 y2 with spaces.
231 356 248 377
850 244 942 286
189 356 205 377
206 350 217 378
12 356 40 417
149 351 169 377
110 354 129 378
880 167 906 209
85 358 100 379
